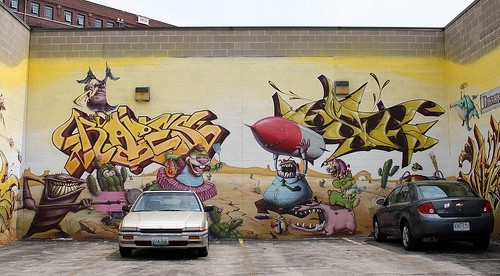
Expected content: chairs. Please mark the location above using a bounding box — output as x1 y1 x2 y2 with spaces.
180 201 195 210
144 201 161 210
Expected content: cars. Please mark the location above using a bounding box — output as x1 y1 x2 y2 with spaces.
373 180 493 251
118 191 213 257
